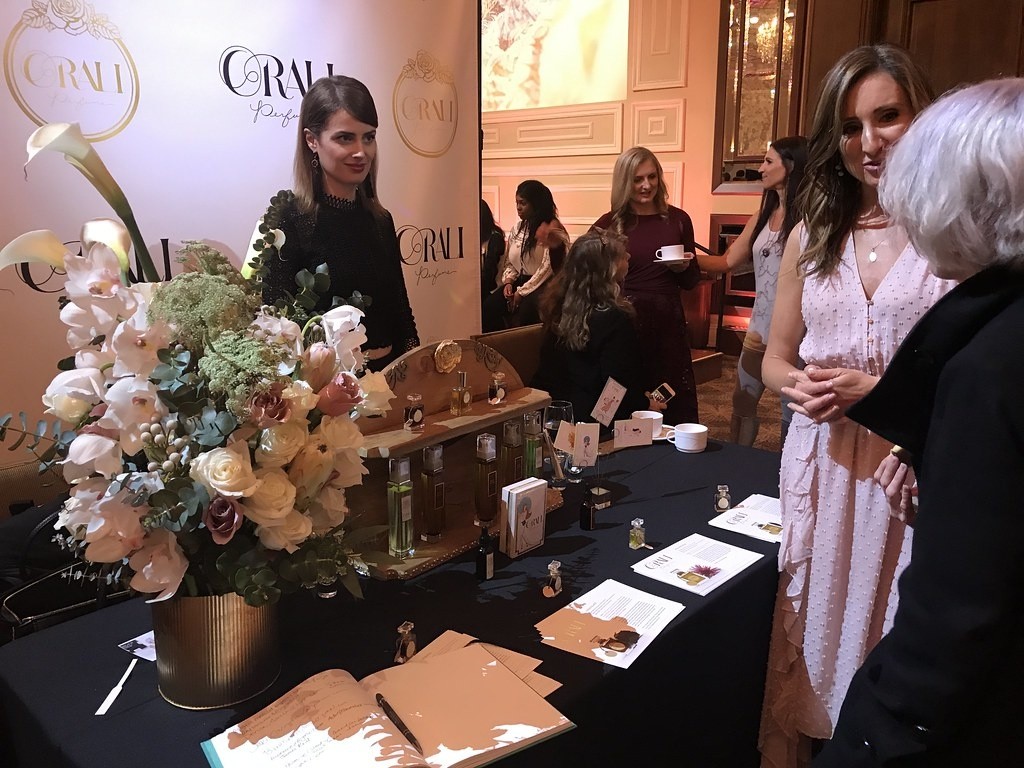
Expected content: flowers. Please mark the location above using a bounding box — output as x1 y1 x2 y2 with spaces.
1 121 396 608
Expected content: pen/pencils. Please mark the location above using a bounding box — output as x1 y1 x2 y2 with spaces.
376 693 424 755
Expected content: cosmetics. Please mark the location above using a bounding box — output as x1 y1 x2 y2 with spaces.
629 518 646 549
394 621 414 663
386 456 416 560
450 371 472 415
404 393 425 431
488 372 508 404
714 485 731 511
475 410 593 598
418 444 446 543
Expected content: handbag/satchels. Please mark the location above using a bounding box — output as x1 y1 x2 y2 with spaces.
512 275 532 294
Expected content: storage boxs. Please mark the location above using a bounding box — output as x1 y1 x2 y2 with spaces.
500 476 548 559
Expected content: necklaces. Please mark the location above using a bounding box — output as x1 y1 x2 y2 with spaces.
763 213 784 257
861 229 894 263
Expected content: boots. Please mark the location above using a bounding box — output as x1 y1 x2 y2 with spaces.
780 419 790 452
729 413 760 447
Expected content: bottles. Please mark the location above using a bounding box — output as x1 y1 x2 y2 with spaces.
580 483 596 530
404 393 425 431
524 410 544 479
474 433 498 527
395 621 417 662
714 484 731 512
546 560 563 597
589 635 628 653
751 521 783 535
488 372 508 404
671 567 708 585
450 371 472 415
387 458 413 559
421 444 448 543
475 527 494 580
501 420 523 486
629 518 645 550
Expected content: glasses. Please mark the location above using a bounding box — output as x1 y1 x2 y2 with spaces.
589 224 609 250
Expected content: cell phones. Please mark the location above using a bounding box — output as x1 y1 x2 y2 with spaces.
650 382 674 404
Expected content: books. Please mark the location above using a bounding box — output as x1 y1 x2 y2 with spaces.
200 645 576 768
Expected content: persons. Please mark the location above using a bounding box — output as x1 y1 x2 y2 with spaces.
758 42 958 768
532 230 666 438
251 76 420 386
502 179 571 327
480 196 504 333
696 136 806 451
589 146 701 424
828 78 1024 768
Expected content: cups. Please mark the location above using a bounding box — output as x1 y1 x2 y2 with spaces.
632 411 664 437
655 244 684 260
666 423 708 453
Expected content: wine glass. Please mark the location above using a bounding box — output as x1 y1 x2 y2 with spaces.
544 400 574 464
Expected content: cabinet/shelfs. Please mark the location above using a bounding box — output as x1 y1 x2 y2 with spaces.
344 339 551 581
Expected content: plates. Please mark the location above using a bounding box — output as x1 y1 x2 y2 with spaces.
652 424 675 440
653 258 692 264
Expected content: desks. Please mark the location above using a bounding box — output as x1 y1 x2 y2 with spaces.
0 435 782 768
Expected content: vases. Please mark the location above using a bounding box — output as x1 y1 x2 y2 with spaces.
152 593 283 711
